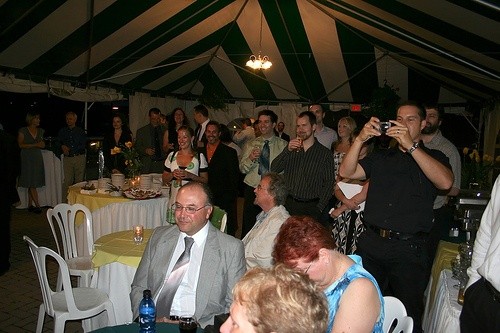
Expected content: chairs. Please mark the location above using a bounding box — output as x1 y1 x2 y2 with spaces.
210 205 228 234
382 296 414 333
46 202 95 292
23 236 117 333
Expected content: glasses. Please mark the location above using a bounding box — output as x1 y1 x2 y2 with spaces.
171 203 206 214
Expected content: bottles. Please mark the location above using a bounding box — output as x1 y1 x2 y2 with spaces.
139 290 157 333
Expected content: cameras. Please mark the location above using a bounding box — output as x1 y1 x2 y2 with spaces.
375 122 392 131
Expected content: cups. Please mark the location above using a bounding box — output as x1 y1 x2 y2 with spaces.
254 144 262 161
178 315 197 333
295 135 303 152
451 243 472 304
132 224 144 245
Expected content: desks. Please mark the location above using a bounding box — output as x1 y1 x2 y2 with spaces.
16 147 64 210
82 228 154 333
420 240 470 333
87 322 204 333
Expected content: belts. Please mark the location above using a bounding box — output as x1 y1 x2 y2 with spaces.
171 316 193 323
370 225 419 240
482 277 500 305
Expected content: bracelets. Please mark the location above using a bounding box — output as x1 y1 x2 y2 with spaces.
408 141 418 153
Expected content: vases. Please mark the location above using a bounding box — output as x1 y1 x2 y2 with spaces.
466 175 491 190
129 169 138 186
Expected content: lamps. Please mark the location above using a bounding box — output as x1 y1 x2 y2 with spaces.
245 13 274 70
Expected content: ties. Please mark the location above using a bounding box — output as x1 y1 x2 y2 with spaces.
156 236 194 318
258 140 270 176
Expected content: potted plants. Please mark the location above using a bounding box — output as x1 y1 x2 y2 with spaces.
104 182 122 197
80 183 98 195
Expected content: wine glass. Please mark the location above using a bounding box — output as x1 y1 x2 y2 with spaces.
178 163 189 171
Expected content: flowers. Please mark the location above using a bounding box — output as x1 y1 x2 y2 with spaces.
110 141 144 173
354 81 401 111
463 147 500 182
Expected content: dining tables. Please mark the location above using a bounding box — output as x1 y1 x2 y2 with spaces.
67 176 170 256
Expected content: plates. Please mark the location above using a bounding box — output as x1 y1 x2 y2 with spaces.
123 189 148 200
90 173 163 193
142 190 157 199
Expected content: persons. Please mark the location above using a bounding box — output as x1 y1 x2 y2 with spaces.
135 104 212 173
102 115 132 179
0 124 21 277
241 172 291 271
330 102 460 333
220 263 329 333
459 173 500 333
234 104 339 240
18 111 45 214
131 181 247 329
57 112 91 203
162 120 239 236
271 216 384 333
